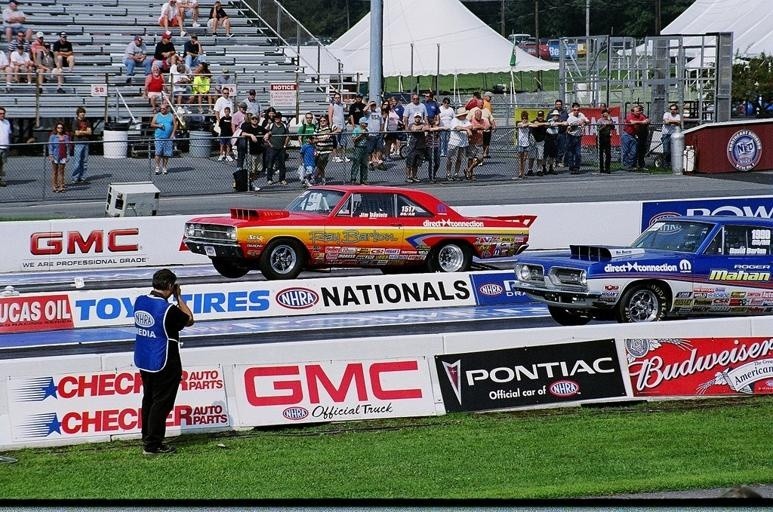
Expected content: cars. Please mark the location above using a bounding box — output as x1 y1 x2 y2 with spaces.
178 185 536 276
510 215 772 324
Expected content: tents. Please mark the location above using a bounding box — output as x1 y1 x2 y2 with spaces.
325 1 560 107
270 46 369 83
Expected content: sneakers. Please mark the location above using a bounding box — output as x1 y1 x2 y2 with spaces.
193 22 201 27
183 107 193 114
155 166 161 174
162 165 169 174
207 107 215 114
252 185 261 192
165 31 172 36
404 177 413 183
332 157 343 163
181 30 188 37
176 106 186 114
142 444 177 455
278 179 287 185
471 173 477 181
267 179 274 185
463 169 471 180
453 176 461 181
342 156 351 162
536 170 543 176
226 155 234 162
212 32 218 37
526 170 534 176
217 155 224 161
446 176 453 181
197 106 205 114
227 33 235 37
412 176 421 183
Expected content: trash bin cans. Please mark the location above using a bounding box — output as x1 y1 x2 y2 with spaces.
102 122 129 159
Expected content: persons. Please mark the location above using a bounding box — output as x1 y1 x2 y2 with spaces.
1 0 75 94
151 104 178 175
597 102 682 174
48 107 92 193
123 31 214 114
297 91 496 193
212 67 291 192
0 108 12 187
516 100 589 179
92 118 104 155
132 269 193 457
158 1 235 40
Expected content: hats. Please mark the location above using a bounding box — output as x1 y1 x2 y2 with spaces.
550 109 561 116
36 31 44 39
250 114 259 120
413 112 422 118
249 89 256 95
359 117 370 124
191 33 198 39
134 35 144 41
638 105 644 109
356 94 363 99
162 32 171 39
455 107 469 117
16 44 24 50
484 91 495 98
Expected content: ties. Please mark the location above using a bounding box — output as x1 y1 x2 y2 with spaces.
573 109 580 111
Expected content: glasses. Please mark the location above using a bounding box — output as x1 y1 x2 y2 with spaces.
320 120 327 122
160 108 166 110
670 108 677 111
18 35 24 38
39 37 43 39
522 118 528 120
224 93 229 94
61 36 67 38
306 117 313 119
0 112 5 115
57 127 64 129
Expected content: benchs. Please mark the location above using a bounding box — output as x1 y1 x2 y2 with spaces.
128 136 182 157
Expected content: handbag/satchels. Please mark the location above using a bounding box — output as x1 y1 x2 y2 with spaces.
333 135 338 150
